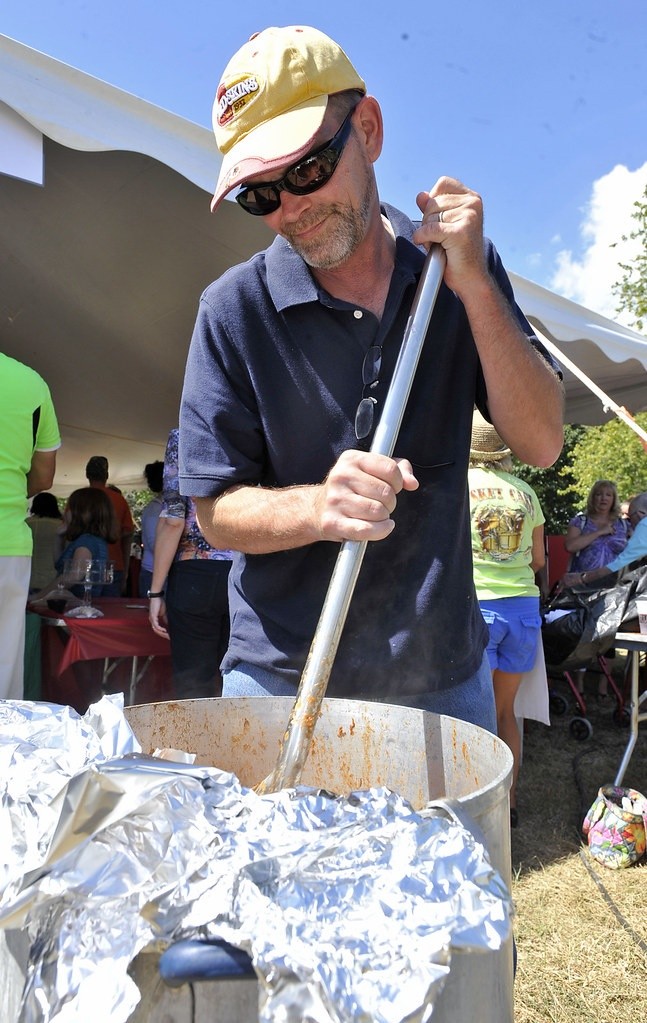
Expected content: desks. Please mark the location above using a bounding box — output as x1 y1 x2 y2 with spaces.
28 586 178 713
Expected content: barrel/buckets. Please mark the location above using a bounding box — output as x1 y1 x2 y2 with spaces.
126 695 517 1023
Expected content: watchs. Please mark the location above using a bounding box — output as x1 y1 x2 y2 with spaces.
147 590 165 599
580 572 591 587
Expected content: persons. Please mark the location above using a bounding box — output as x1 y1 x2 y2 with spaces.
562 495 647 589
27 487 119 604
466 409 546 827
175 25 566 736
62 456 134 597
566 479 635 705
139 461 169 598
148 428 233 699
0 351 61 700
24 492 62 595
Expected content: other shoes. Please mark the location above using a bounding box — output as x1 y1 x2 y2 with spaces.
575 692 615 711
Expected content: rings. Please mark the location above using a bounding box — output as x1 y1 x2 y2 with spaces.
151 626 155 629
438 210 445 222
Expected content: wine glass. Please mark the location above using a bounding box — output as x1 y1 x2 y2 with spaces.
60 556 115 618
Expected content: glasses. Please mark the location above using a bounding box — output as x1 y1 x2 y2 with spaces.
234 105 359 217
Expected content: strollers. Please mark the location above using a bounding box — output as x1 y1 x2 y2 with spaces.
538 575 631 742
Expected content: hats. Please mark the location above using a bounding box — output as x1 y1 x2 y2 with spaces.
468 408 512 463
209 24 367 215
